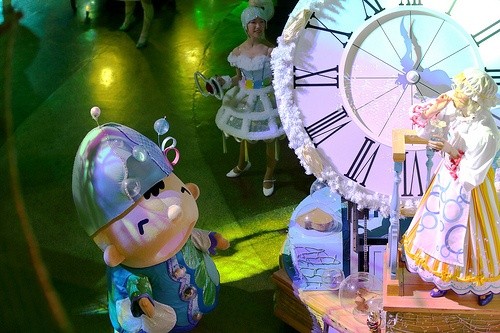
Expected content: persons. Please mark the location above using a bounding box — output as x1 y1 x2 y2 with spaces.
120 0 155 48
402 69 500 306
216 0 286 196
71 107 231 333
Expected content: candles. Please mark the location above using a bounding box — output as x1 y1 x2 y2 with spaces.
270 0 500 220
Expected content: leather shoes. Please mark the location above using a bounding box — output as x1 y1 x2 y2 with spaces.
226 161 251 177
262 175 276 196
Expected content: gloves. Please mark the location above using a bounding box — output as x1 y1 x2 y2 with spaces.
206 74 231 95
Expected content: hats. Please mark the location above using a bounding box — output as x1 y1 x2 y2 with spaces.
242 7 267 32
